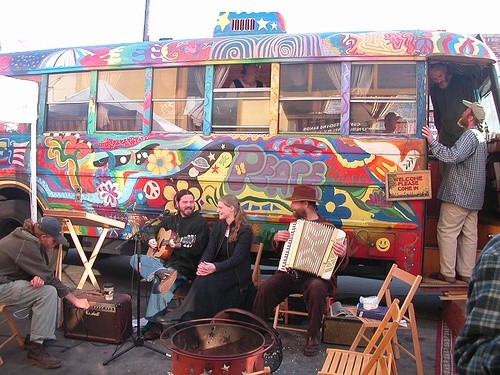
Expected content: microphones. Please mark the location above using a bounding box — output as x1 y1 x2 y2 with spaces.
158 208 170 222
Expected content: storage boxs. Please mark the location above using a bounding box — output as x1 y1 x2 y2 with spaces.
321 304 375 348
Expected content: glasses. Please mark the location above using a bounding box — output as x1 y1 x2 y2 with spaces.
255 64 264 69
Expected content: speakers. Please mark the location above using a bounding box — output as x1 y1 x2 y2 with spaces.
62 286 133 346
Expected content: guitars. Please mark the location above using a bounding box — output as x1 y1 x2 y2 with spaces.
146 227 196 259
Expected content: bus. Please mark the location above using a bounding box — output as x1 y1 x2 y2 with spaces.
0 9 500 292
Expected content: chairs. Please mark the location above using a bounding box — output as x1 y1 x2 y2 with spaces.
346 263 423 375
0 304 26 366
317 298 401 375
249 242 265 287
274 293 310 333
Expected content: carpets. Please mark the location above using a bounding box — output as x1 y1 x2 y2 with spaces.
435 319 457 375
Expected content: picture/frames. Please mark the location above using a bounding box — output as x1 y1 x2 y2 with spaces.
385 170 432 201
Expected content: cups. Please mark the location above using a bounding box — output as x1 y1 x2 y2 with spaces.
103 283 114 301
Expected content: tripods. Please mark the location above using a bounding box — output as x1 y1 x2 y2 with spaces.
101 238 172 366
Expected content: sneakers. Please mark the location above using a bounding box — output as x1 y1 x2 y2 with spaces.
430 273 456 283
24 334 31 350
27 342 62 369
456 276 471 283
155 269 178 293
144 323 163 339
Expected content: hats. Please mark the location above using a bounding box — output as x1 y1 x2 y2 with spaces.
385 112 402 121
462 100 485 123
283 186 323 201
36 217 67 244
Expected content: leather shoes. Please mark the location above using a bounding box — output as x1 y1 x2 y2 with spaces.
304 335 320 357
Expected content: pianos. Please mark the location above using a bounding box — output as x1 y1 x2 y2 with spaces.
278 218 346 280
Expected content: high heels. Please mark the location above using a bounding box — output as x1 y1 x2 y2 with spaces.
156 314 179 325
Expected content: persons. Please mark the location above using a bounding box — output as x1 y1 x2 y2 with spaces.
0 217 90 368
129 189 209 340
282 63 321 114
452 232 500 375
421 100 487 284
249 185 350 357
428 63 475 180
224 63 267 125
156 196 252 326
